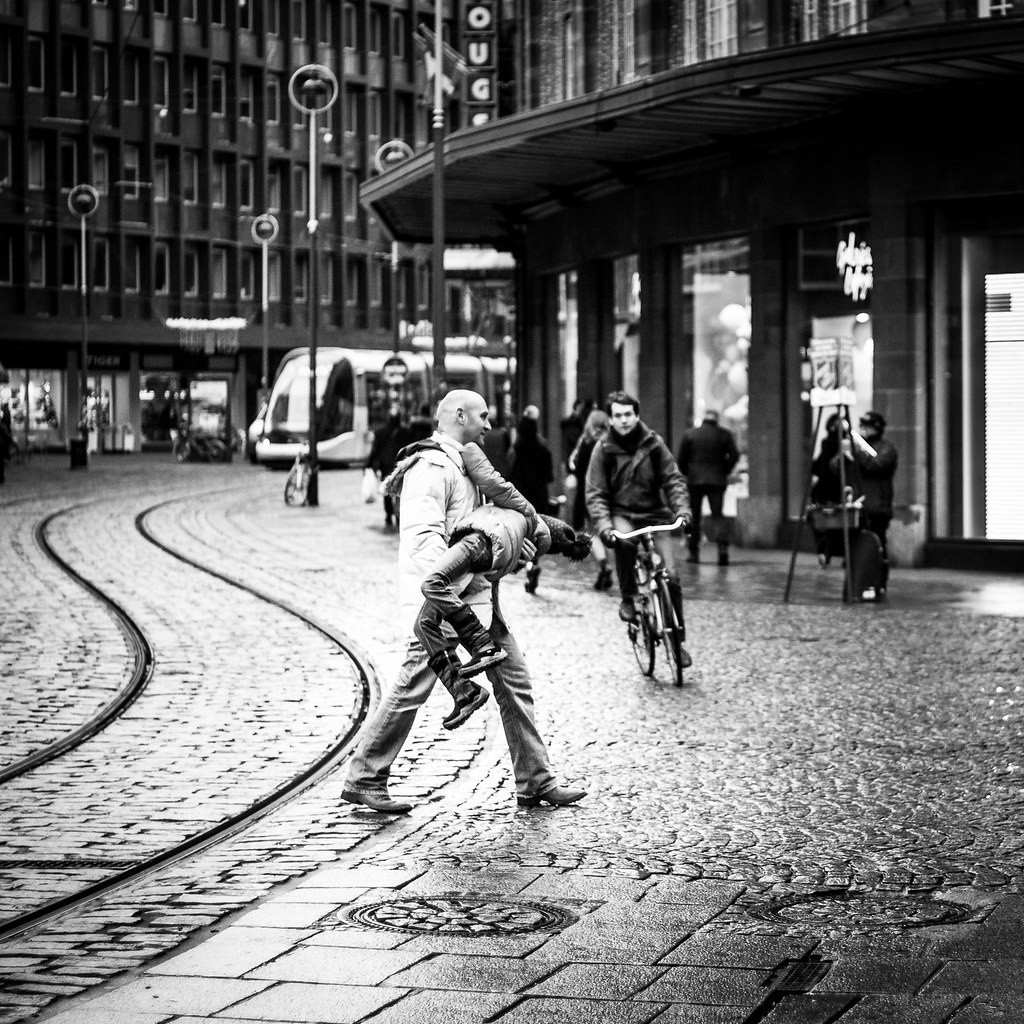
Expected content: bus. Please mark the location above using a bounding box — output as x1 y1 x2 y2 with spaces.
250 335 520 473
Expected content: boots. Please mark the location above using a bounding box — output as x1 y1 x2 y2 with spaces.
442 603 510 678
427 646 490 730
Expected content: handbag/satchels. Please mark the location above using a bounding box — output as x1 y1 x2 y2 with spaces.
362 467 382 504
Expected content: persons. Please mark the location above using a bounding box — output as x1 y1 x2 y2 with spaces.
840 412 899 601
505 405 554 518
341 389 587 812
586 392 692 669
808 414 859 566
675 410 741 566
569 410 615 536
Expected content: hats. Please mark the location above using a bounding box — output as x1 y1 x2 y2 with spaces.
860 411 888 436
540 513 594 561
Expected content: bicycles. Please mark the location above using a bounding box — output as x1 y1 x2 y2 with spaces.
281 439 314 508
604 513 693 688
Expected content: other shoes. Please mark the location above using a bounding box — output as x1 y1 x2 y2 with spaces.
717 554 730 567
860 585 887 603
619 599 636 623
385 515 393 525
675 642 692 669
517 787 588 807
595 565 614 591
526 566 540 592
685 555 700 564
341 788 412 814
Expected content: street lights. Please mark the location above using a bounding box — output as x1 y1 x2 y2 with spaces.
67 183 99 422
373 141 416 354
249 214 280 398
286 61 340 498
